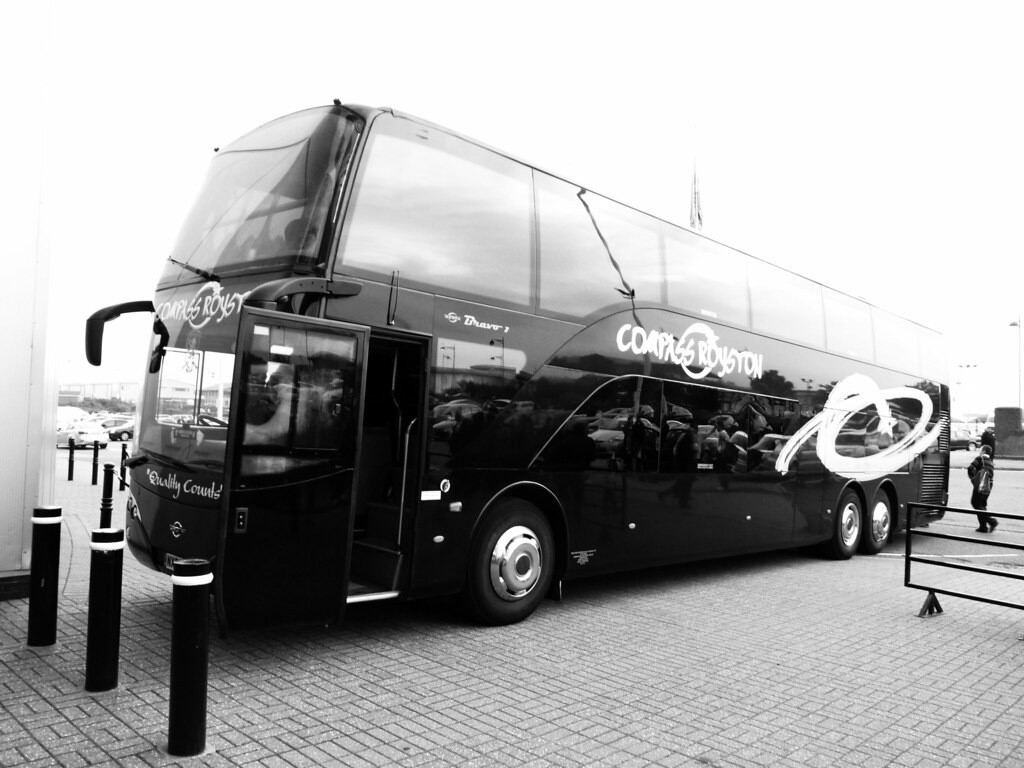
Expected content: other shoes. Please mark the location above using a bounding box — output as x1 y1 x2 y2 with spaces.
976 527 987 532
988 519 998 532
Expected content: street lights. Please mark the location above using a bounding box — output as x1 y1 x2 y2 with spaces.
1008 319 1021 412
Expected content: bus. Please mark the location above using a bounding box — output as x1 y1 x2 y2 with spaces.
84 99 956 635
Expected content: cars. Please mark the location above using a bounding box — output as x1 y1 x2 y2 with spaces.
948 427 984 451
55 405 194 450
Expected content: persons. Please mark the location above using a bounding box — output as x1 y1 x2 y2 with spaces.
981 427 996 461
621 405 740 508
446 405 498 464
967 445 999 533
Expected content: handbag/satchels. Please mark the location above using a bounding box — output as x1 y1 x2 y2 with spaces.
972 456 991 495
